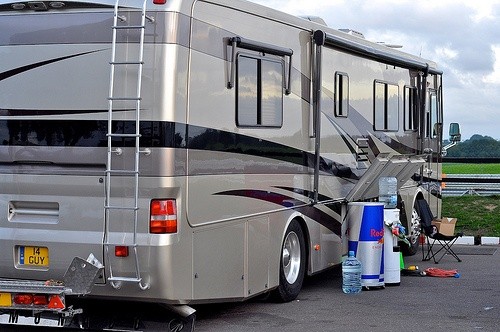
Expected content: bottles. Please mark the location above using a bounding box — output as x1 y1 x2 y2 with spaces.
342 251 363 294
407 266 419 270
379 176 397 209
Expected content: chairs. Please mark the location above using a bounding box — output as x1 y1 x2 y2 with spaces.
416 199 463 264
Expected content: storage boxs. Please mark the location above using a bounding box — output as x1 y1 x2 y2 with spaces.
432 217 457 236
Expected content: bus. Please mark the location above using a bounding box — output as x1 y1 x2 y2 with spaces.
0 0 462 332
